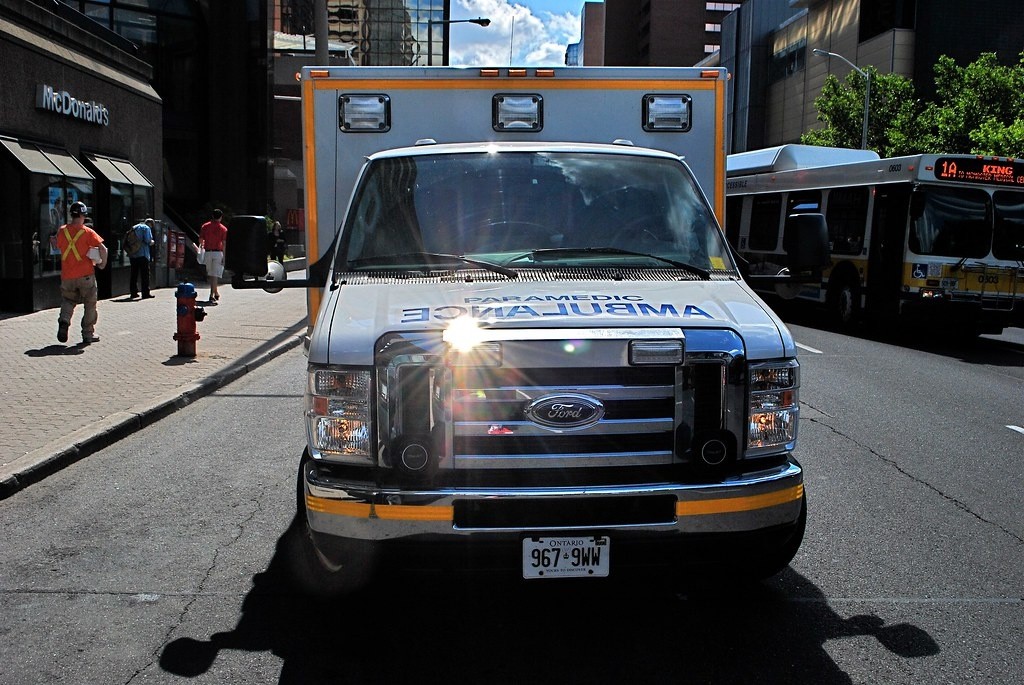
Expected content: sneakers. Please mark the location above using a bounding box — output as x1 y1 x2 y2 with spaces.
208 298 218 306
215 295 220 300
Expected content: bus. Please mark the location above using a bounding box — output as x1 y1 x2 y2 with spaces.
725 141 1024 342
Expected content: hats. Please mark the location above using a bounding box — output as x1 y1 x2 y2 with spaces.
70 202 88 214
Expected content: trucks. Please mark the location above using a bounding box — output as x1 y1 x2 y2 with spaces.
225 66 830 611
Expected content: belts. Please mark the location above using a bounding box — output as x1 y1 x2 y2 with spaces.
205 250 223 252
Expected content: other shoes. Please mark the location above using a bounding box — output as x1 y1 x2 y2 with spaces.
131 293 139 298
82 337 100 345
142 294 155 299
57 319 68 343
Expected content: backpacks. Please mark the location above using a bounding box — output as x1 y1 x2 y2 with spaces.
122 226 145 255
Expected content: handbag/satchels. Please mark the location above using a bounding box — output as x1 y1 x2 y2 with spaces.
197 243 205 264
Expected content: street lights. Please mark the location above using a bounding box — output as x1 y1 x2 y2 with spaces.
812 49 870 150
427 17 491 67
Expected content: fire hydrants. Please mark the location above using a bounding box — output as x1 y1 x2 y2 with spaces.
172 277 207 357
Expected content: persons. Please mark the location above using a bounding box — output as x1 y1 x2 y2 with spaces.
128 217 156 298
266 221 288 265
199 209 228 305
55 201 108 343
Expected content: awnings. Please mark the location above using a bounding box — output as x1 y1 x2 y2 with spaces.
82 151 155 188
0 135 96 180
